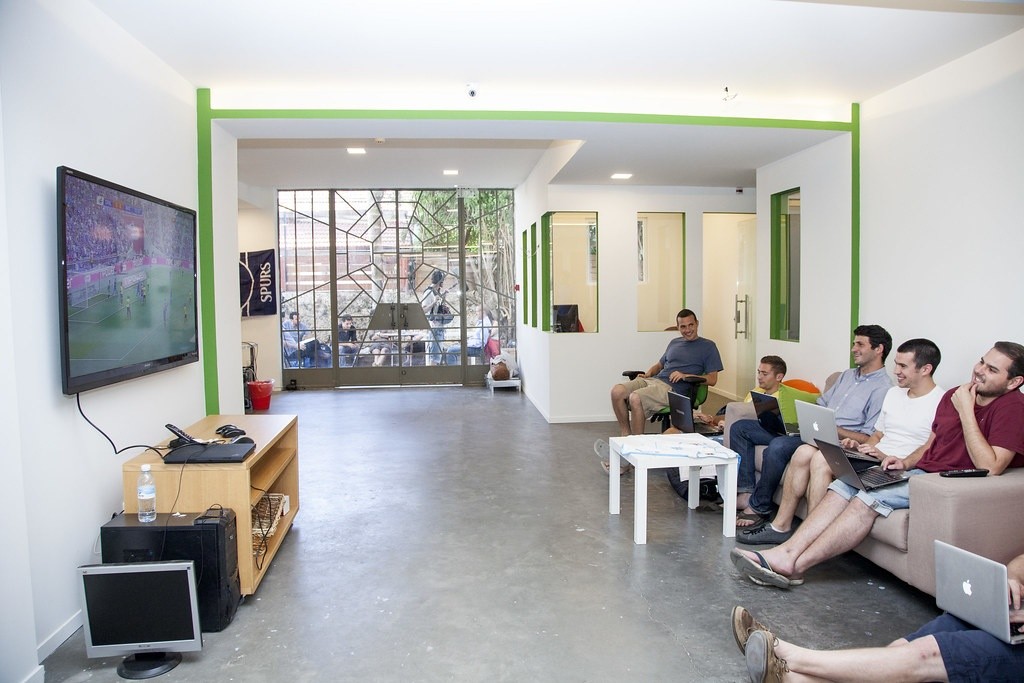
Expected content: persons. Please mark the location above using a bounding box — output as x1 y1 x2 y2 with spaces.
729 342 1024 589
65 174 194 322
663 355 786 434
421 271 447 365
594 309 724 475
736 339 946 545
369 330 392 366
445 305 493 364
329 314 360 367
731 554 1024 683
282 312 332 368
730 325 894 531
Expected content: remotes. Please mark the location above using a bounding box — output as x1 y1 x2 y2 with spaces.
941 468 989 478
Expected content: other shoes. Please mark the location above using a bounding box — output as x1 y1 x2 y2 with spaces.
320 353 331 359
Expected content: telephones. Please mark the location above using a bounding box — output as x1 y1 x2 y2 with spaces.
165 424 194 447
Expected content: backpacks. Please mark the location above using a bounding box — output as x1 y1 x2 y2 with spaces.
436 302 453 324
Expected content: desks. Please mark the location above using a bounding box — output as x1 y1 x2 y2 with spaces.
242 343 257 382
489 378 521 394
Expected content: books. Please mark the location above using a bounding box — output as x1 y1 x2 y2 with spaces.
164 443 256 464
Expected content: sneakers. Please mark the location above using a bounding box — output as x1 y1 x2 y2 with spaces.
745 630 789 683
738 522 793 545
731 605 779 657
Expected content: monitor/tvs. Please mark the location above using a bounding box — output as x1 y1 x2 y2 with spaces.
77 560 204 681
554 305 579 332
56 166 199 395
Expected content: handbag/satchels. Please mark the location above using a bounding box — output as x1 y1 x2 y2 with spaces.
668 468 720 501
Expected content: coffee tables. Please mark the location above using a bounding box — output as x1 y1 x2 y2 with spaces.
609 433 738 544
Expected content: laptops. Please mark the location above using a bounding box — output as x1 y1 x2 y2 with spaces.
750 390 800 438
934 540 1024 644
794 400 880 463
667 391 724 436
814 439 914 492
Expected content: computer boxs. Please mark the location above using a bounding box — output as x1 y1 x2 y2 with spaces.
100 506 242 634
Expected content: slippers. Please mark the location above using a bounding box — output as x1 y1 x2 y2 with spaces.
748 574 803 585
594 440 630 467
601 461 628 475
730 548 790 588
736 511 775 529
695 502 743 513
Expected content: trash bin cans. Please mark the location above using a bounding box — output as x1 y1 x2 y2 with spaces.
247 380 273 410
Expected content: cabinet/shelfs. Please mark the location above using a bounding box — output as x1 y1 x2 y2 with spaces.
122 414 300 595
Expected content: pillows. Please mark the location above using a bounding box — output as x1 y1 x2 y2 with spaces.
778 382 821 432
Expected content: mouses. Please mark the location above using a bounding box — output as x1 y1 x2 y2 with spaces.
216 424 254 444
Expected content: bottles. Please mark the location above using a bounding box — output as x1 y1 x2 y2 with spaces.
137 464 157 522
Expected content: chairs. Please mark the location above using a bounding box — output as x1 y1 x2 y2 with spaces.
622 371 708 434
282 329 491 369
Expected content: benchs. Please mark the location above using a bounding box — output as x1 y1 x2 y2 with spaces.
723 371 1024 598
